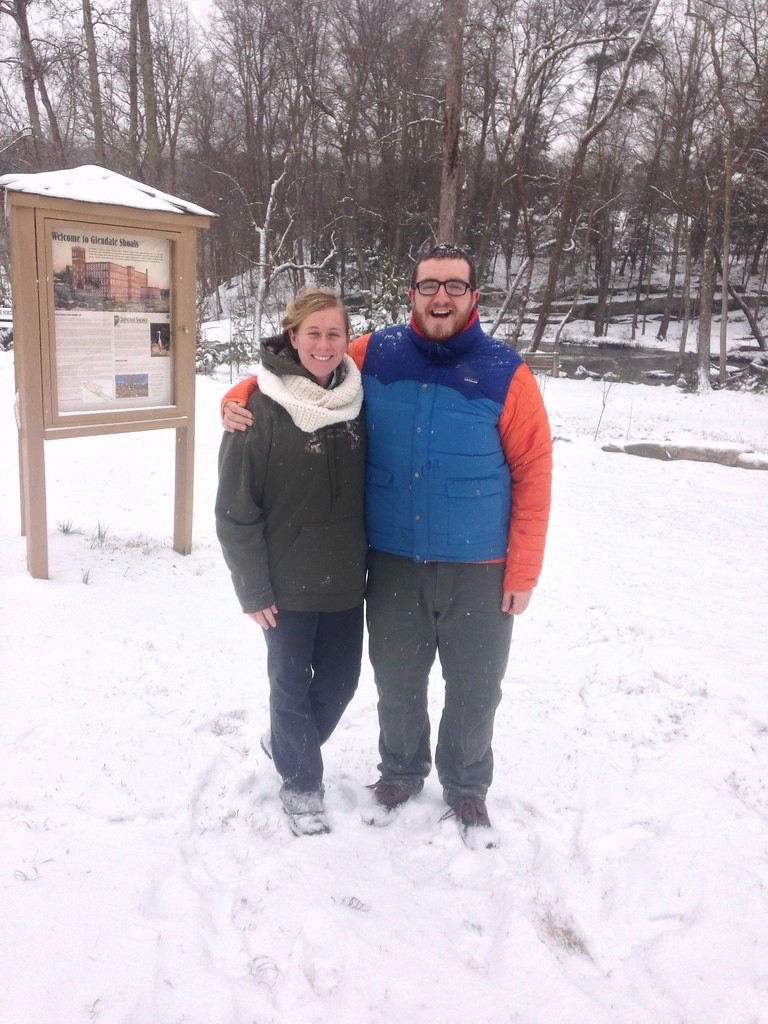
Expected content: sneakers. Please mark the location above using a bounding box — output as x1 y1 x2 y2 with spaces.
260 730 277 759
438 783 496 851
279 779 330 836
360 774 424 827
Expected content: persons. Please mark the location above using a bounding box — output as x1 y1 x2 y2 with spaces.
219 243 554 849
214 290 368 837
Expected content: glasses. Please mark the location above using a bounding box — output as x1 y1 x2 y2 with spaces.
414 279 474 296
